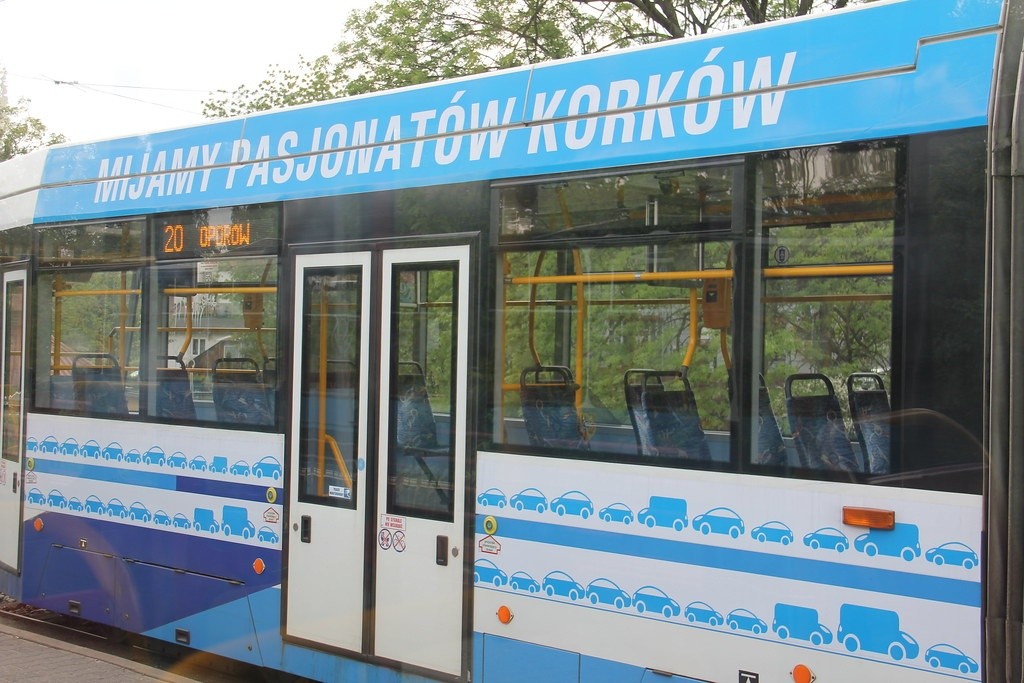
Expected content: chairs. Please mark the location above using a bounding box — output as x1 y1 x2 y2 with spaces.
728 373 891 477
318 358 450 510
518 366 589 450
72 352 277 429
624 368 712 462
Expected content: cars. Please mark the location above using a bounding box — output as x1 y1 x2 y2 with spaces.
861 366 890 390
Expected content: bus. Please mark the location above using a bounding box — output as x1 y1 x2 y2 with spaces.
0 0 1024 682
